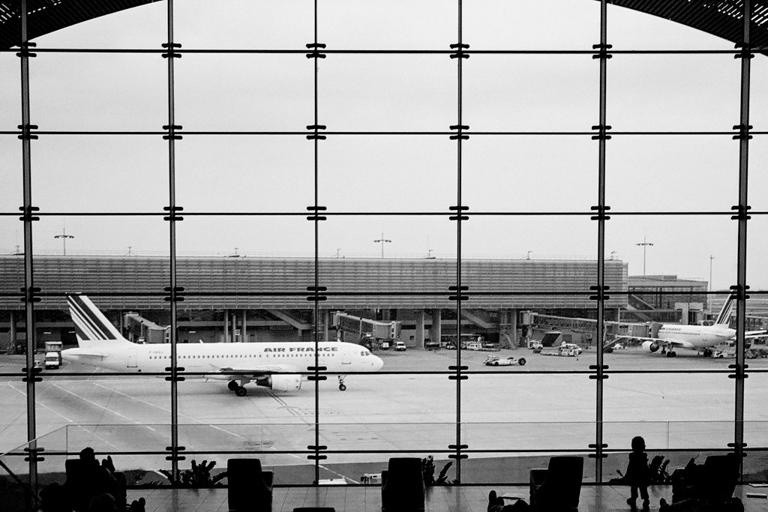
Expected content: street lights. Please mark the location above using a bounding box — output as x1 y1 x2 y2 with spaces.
636 237 655 276
709 254 715 291
373 233 392 258
53 226 75 256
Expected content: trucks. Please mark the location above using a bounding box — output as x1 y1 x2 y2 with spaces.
360 472 382 484
44 341 63 369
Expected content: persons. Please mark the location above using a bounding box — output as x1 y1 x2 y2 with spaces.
620 435 652 508
80 447 117 487
486 485 543 510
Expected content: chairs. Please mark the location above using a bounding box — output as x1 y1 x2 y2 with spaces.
227 459 273 512
530 457 583 512
65 460 117 512
672 455 740 512
382 458 425 512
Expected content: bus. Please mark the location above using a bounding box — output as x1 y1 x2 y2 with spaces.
441 333 481 348
714 340 736 356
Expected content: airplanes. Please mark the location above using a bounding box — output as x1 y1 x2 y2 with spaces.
61 291 384 396
614 285 768 358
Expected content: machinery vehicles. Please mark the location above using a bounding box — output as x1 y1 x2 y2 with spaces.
710 346 728 358
530 331 583 356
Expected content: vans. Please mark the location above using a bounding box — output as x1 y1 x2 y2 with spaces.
393 341 407 351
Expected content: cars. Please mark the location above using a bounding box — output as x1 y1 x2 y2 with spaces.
312 477 348 484
34 358 42 373
